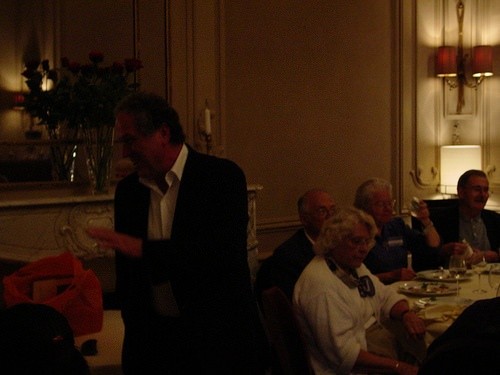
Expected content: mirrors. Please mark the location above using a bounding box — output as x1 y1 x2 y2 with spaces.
0 0 173 192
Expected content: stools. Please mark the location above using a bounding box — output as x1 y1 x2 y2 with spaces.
71 309 127 375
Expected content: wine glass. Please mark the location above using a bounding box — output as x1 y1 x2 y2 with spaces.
448 255 467 302
471 254 488 294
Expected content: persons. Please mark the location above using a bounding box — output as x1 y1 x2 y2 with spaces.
294 208 420 375
86 91 267 375
258 190 339 375
418 296 500 375
354 178 440 286
423 169 500 271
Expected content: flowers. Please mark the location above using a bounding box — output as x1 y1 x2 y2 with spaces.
48 52 139 131
21 56 74 133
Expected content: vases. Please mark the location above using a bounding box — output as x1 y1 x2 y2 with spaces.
81 129 116 194
52 125 79 181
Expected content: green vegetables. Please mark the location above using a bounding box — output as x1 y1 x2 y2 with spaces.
422 281 438 289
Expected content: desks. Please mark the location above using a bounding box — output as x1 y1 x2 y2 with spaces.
372 260 499 362
1 187 265 278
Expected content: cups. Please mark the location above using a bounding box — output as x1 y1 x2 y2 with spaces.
488 264 500 298
409 194 422 218
400 251 412 269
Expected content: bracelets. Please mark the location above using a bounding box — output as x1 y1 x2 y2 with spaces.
395 362 399 374
421 219 433 234
399 310 416 322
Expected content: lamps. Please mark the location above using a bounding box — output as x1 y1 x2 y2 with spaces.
438 145 487 197
431 40 498 93
12 90 26 108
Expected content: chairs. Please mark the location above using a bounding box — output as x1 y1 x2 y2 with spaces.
258 286 319 374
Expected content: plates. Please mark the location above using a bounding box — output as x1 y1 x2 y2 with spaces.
415 269 475 282
412 297 474 310
397 282 461 295
472 263 500 273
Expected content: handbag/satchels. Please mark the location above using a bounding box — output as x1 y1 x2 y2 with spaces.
2 254 104 337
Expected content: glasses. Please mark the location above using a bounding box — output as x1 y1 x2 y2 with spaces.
306 206 339 219
374 200 396 208
348 237 376 247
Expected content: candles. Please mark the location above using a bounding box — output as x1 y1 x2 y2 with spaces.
204 109 213 131
